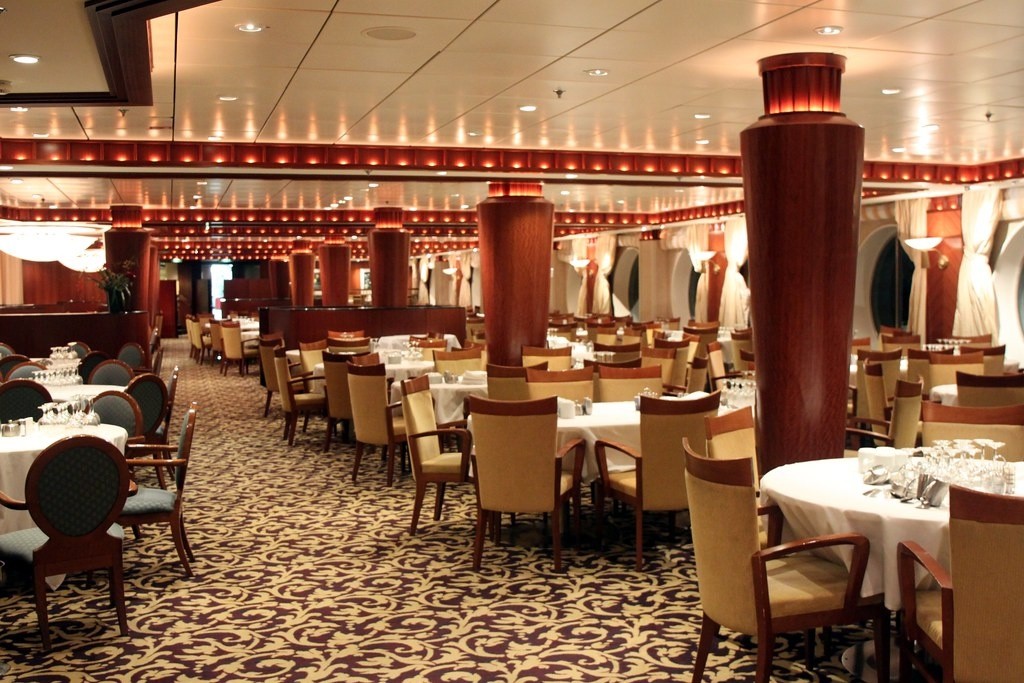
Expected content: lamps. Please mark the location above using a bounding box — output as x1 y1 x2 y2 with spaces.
905 236 949 269
694 250 720 274
0 219 111 263
57 234 105 274
570 256 593 277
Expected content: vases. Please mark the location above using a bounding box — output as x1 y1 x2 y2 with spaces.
108 286 132 315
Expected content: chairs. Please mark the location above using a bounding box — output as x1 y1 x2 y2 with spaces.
0 433 139 652
401 376 498 543
846 324 1023 463
595 389 722 572
0 325 165 411
123 373 169 490
115 401 197 577
185 310 260 377
83 391 145 479
468 394 586 571
897 483 1024 683
680 436 891 683
155 365 178 481
346 360 412 488
0 378 53 425
255 312 486 454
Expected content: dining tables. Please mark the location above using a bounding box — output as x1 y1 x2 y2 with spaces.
390 379 489 473
760 456 1024 683
42 385 128 402
468 401 732 544
486 310 755 422
927 382 958 406
285 349 400 364
313 360 435 418
0 422 128 592
848 359 908 417
28 356 82 372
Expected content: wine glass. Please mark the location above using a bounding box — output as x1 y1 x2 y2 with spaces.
34 342 83 393
39 394 100 436
719 378 756 410
928 438 1008 495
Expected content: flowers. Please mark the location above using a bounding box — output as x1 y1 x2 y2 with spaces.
78 259 137 291
